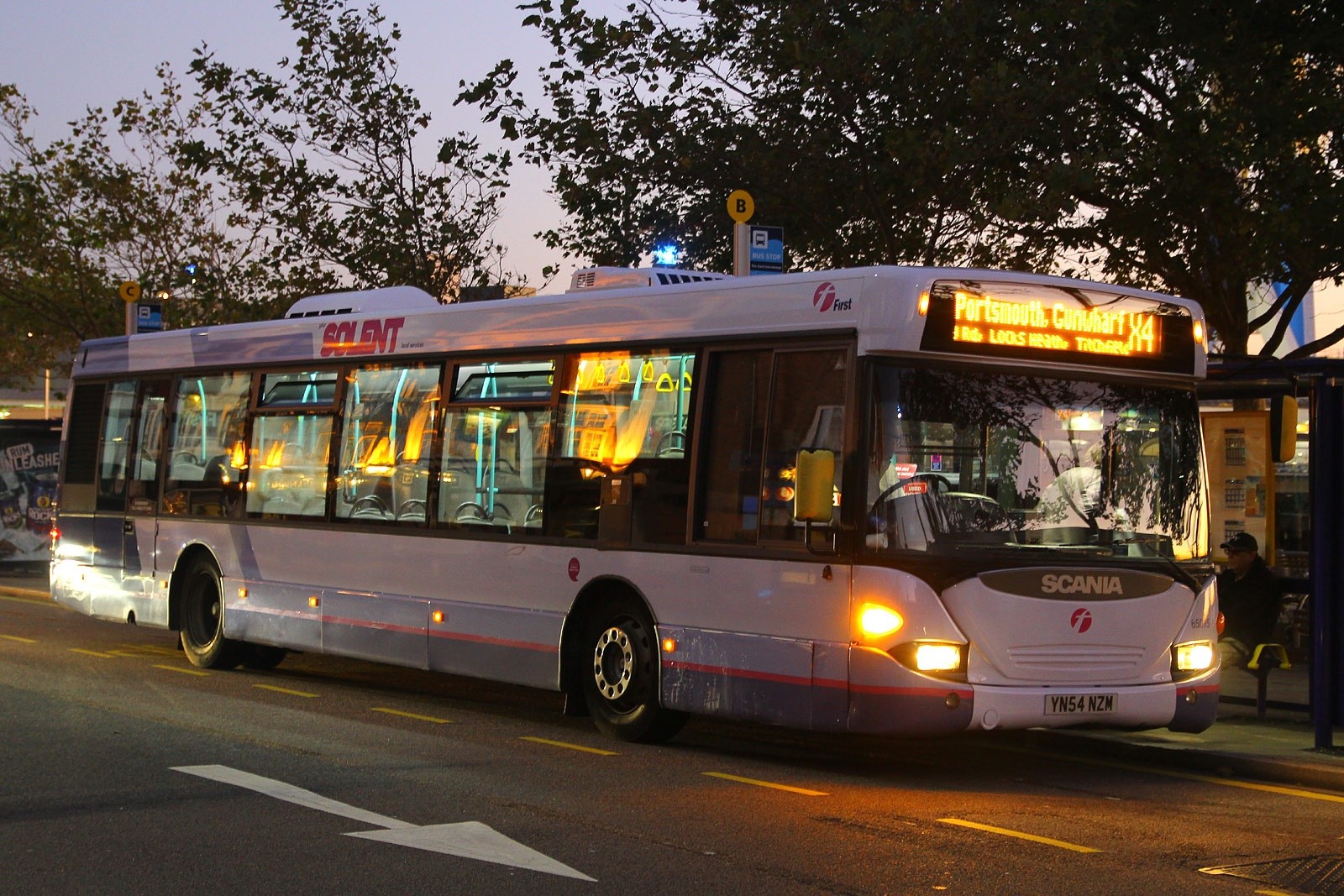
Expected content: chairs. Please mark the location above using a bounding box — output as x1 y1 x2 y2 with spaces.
120 432 686 546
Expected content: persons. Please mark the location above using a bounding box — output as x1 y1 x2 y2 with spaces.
1215 532 1283 671
1029 438 1134 533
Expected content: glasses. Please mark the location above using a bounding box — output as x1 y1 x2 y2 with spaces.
1224 549 1245 556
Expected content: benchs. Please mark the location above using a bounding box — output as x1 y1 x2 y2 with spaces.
1220 578 1313 715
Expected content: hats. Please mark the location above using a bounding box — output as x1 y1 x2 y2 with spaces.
1220 532 1258 551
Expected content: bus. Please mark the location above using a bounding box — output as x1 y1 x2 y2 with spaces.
49 265 1298 743
0 399 67 561
